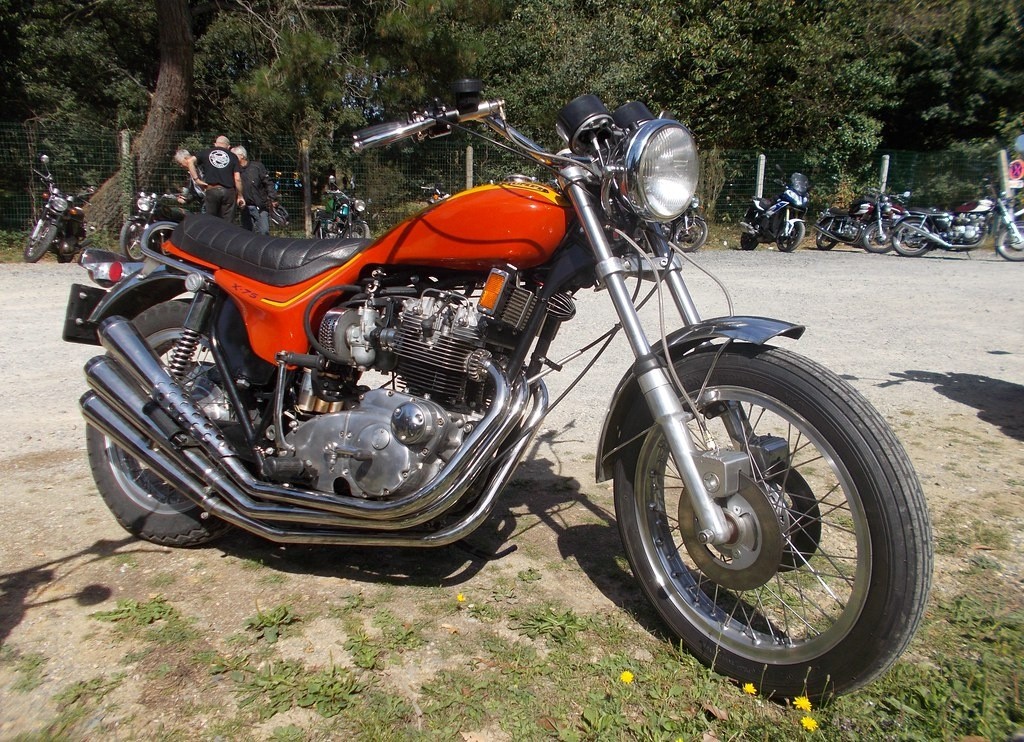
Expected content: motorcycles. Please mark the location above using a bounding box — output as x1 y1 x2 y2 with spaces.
24 155 98 263
737 163 814 253
119 188 200 263
419 184 451 205
891 174 1024 262
660 193 708 252
64 72 933 706
811 176 928 253
311 175 370 240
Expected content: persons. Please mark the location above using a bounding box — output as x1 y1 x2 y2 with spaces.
175 135 278 236
